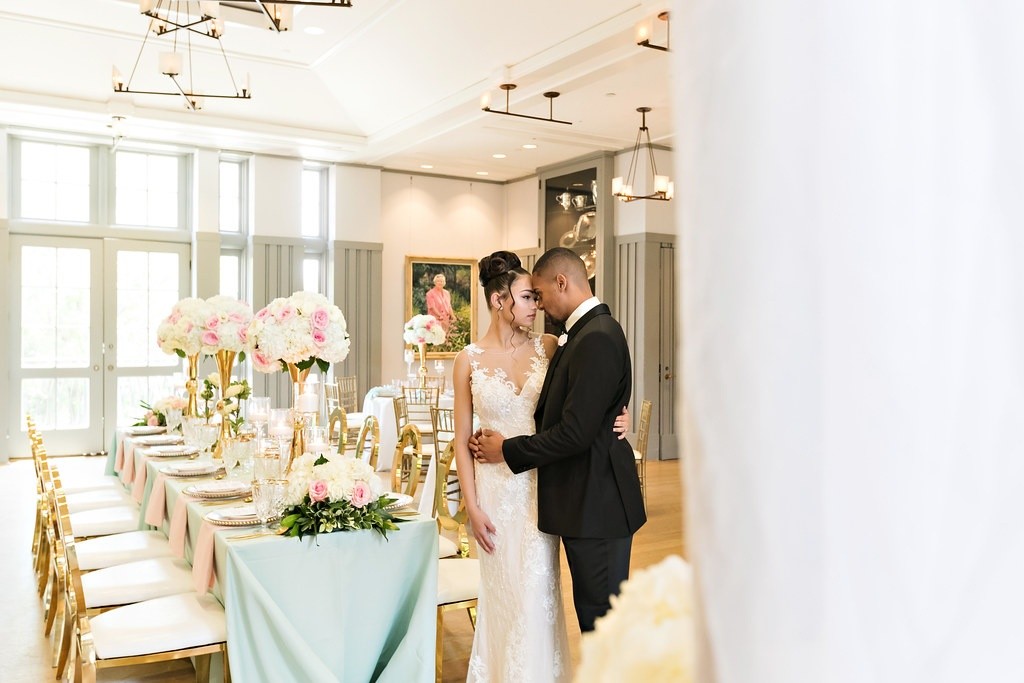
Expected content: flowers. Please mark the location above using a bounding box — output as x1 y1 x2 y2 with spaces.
403 314 445 345
129 372 251 436
275 451 413 546
157 291 359 360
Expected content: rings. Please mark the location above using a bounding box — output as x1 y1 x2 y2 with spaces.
623 429 625 432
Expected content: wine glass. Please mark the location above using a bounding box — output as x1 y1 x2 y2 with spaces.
166 397 328 531
391 381 439 399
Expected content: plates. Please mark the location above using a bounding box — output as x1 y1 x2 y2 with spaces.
123 426 413 526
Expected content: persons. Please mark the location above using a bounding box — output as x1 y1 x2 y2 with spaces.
453 247 647 683
426 274 457 344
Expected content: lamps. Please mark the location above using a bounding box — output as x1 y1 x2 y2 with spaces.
555 179 597 210
637 1 674 54
113 0 355 112
481 74 572 124
609 106 675 202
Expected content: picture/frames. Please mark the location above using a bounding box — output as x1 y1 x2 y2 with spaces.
405 255 477 360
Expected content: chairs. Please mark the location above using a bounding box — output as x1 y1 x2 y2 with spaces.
324 375 683 683
16 410 232 683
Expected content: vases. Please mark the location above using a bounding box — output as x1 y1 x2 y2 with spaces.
182 349 202 416
286 359 313 478
214 348 237 459
417 343 428 388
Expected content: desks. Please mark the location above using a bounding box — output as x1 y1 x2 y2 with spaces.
106 416 439 683
364 382 480 473
